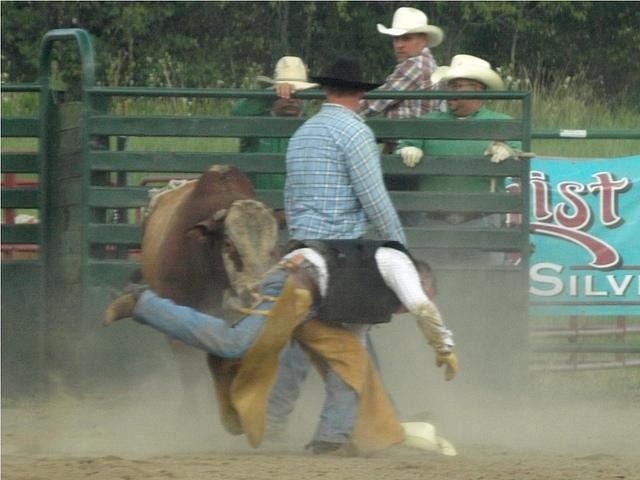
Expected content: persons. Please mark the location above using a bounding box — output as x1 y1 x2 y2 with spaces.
356 6 446 225
102 237 461 458
388 53 525 265
260 56 409 446
226 55 321 228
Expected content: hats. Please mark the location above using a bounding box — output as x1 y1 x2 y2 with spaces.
430 53 507 93
401 421 459 458
309 56 386 92
376 7 445 49
256 55 321 91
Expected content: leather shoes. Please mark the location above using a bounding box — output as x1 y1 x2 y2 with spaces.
102 283 149 327
312 440 357 456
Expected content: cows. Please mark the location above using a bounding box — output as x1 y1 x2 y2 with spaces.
138 163 287 437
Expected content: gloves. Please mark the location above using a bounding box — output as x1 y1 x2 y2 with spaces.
436 353 459 382
484 140 514 164
399 146 423 168
